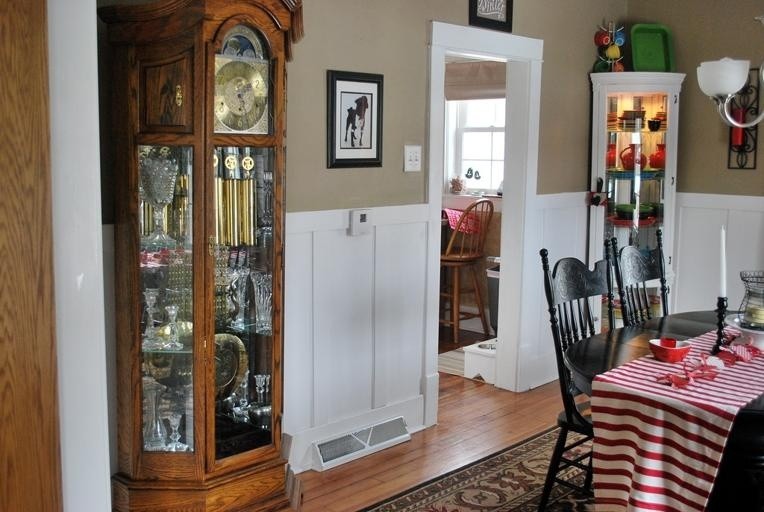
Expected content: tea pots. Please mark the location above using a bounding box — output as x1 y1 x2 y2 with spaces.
607 144 615 167
650 144 665 169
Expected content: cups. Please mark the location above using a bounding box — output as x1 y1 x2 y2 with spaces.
647 120 661 132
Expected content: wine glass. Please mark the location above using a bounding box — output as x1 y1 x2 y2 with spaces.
139 162 179 245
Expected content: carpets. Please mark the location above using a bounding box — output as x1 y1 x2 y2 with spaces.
439 338 486 384
356 426 596 512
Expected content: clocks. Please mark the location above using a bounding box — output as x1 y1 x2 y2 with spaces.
97 0 304 511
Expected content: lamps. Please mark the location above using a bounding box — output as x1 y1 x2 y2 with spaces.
696 14 764 128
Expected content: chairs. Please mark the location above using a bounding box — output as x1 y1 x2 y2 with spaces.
612 230 669 328
439 199 494 343
538 238 613 512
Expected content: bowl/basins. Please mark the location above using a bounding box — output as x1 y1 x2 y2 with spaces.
614 204 654 219
648 338 692 362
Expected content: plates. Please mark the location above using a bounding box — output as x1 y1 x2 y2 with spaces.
617 108 646 131
607 112 616 131
653 111 667 130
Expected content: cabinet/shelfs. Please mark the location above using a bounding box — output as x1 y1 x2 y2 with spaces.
586 72 688 337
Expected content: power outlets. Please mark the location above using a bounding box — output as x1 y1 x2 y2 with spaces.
404 144 422 173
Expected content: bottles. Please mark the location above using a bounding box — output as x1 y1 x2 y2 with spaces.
619 144 647 170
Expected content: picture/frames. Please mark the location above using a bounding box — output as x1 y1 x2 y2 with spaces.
327 72 383 168
469 0 512 32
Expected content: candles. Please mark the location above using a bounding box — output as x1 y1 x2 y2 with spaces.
731 109 746 144
718 226 727 297
